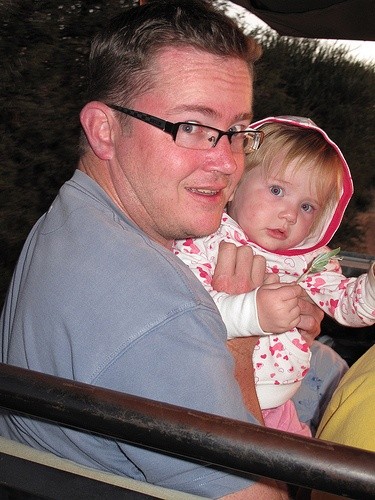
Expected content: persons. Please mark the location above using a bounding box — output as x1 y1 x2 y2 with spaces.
0 0 324 500
298 343 375 500
171 115 375 437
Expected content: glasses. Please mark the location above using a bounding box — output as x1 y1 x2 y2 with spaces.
104 105 265 154
